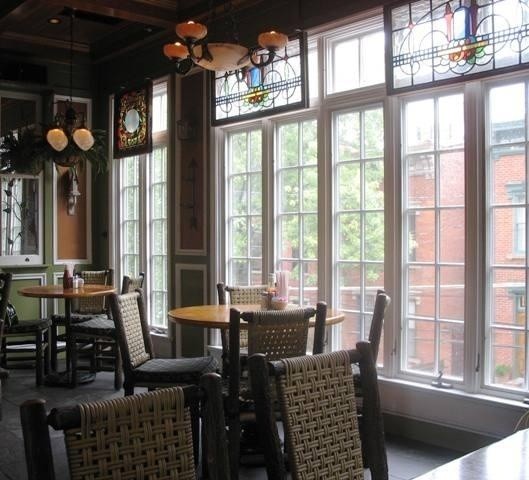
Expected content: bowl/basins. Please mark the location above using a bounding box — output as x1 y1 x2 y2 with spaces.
271 302 287 310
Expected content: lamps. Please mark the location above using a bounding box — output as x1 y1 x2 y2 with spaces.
163 0 287 75
47 9 95 152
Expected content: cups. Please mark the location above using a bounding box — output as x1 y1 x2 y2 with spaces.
79 279 84 288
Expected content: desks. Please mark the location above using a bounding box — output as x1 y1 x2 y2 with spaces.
408 427 529 480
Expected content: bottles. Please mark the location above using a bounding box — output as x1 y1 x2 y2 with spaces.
261 292 268 310
64 270 78 289
